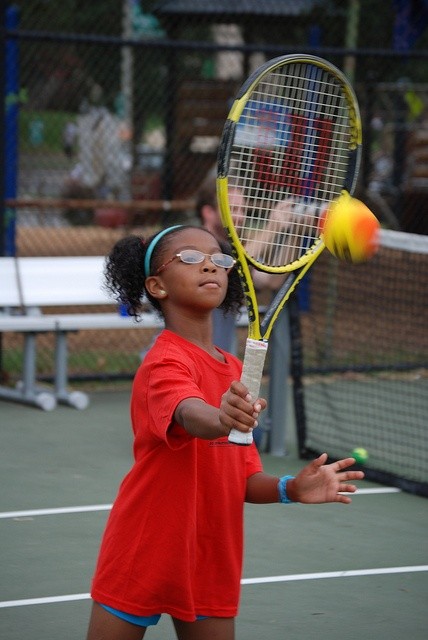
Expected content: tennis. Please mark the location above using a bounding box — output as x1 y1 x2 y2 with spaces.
350 446 369 464
320 197 382 265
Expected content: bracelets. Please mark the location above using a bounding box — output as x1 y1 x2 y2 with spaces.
277 474 296 504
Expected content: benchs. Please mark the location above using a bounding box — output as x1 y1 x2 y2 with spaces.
1 255 271 412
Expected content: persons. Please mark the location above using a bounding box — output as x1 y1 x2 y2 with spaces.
85 223 364 639
193 177 294 359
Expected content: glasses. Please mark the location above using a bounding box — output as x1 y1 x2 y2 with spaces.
154 248 236 274
